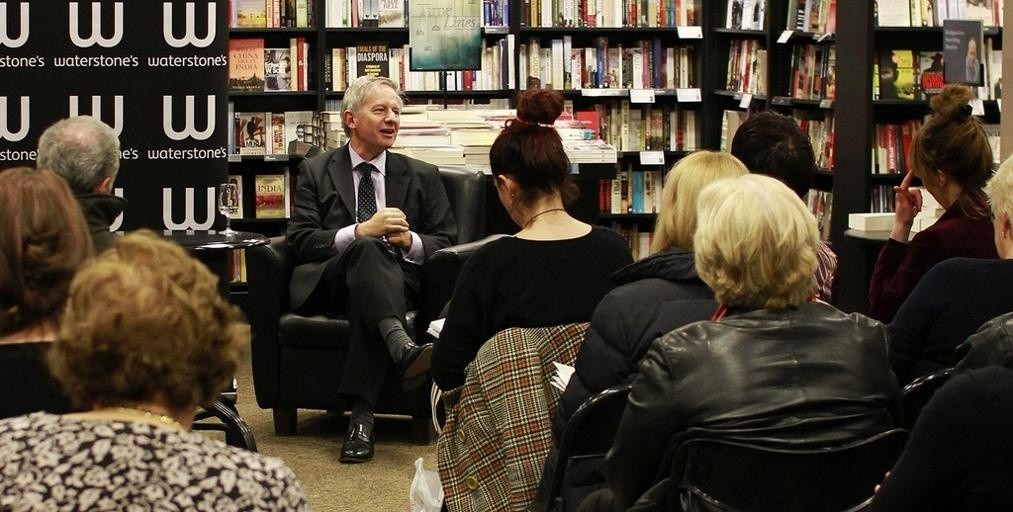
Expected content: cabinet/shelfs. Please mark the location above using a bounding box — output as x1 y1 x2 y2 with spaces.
709 0 872 245
871 1 1000 191
226 0 708 261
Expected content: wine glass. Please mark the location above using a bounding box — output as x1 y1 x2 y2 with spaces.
214 183 240 237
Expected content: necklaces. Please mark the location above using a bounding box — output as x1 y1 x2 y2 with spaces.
93 401 185 427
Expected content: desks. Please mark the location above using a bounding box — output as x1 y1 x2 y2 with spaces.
105 224 270 453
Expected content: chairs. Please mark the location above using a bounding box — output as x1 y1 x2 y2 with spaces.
665 428 912 512
898 367 953 404
242 160 486 449
530 383 630 512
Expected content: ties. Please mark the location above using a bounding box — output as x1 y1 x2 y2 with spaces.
355 161 378 225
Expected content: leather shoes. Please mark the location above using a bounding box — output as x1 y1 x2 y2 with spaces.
336 418 380 465
391 337 437 399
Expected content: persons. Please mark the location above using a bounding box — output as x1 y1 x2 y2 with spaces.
36 115 130 254
1 166 96 419
283 72 459 463
534 148 750 512
0 229 312 512
429 87 634 511
573 175 909 512
